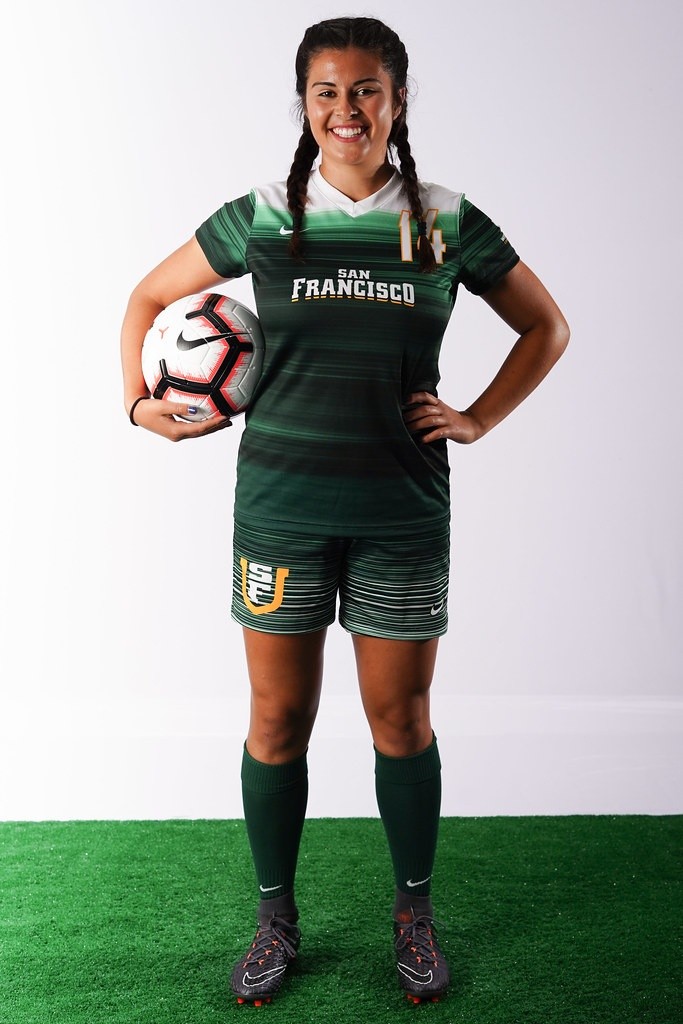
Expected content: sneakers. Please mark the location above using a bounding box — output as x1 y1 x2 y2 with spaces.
229 924 301 1006
393 919 449 1005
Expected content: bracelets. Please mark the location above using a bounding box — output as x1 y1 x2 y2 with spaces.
130 396 150 426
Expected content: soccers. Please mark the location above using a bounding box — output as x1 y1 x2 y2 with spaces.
141 292 263 422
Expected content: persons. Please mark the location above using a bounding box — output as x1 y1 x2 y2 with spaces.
121 18 570 1007
